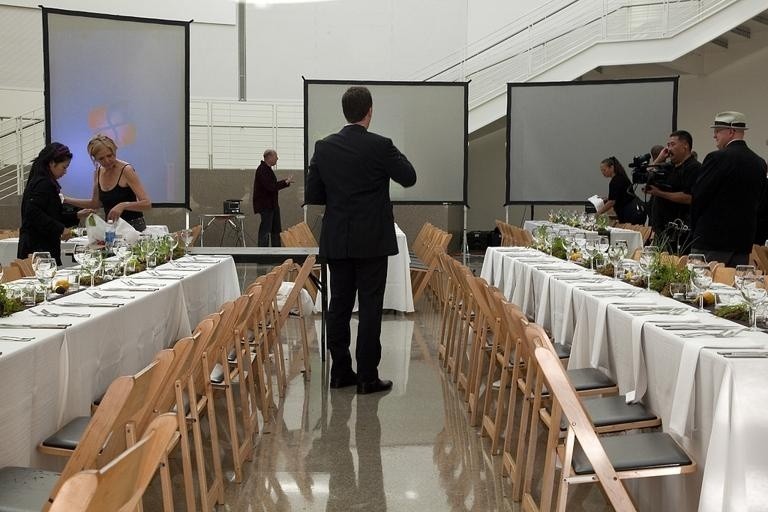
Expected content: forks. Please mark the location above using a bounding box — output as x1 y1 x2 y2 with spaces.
672 330 768 358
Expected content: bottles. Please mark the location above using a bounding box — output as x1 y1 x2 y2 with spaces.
103 219 117 249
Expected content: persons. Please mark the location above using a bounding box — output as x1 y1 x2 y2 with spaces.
16 143 95 263
592 154 643 226
59 131 152 231
647 144 666 227
249 147 297 278
323 388 393 512
638 131 701 260
302 86 417 395
688 107 768 274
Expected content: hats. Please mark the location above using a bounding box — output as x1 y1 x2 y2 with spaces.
709 110 749 130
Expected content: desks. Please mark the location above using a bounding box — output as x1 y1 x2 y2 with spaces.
194 211 246 247
0 246 242 476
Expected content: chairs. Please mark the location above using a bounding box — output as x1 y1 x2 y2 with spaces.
428 242 697 512
0 220 320 509
404 222 454 307
495 209 768 330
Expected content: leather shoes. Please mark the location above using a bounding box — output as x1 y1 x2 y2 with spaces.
330 370 358 390
356 377 393 395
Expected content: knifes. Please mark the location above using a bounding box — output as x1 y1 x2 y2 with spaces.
494 247 531 263
0 323 72 344
196 255 230 263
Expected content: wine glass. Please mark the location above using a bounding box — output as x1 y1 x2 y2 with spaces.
531 222 767 330
0 230 196 316
545 207 607 232
